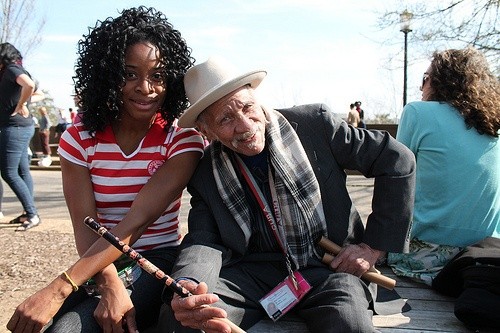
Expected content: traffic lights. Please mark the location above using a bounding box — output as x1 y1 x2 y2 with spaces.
398 8 415 108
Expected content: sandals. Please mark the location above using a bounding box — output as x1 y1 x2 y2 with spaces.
13 216 39 231
10 214 29 223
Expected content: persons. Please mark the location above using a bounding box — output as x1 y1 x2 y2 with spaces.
53 108 67 138
37 106 52 167
6 5 209 333
30 113 39 125
163 55 417 333
377 47 500 286
347 101 364 129
266 301 277 317
69 108 77 125
0 42 40 232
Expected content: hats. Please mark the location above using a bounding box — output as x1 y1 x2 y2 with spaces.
177 57 268 127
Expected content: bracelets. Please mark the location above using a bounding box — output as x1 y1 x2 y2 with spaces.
60 270 79 292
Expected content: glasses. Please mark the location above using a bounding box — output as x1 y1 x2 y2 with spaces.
421 74 432 87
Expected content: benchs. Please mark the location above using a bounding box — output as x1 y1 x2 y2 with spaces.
247 264 471 333
